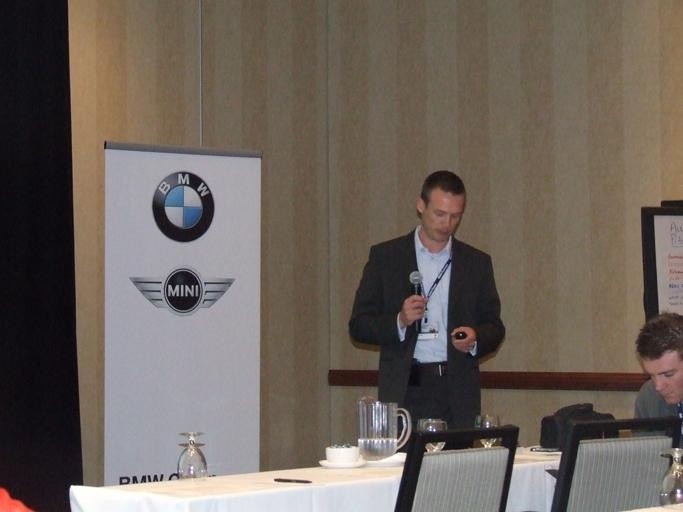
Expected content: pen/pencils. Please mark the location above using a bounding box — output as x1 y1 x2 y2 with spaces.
531 448 560 451
274 477 311 484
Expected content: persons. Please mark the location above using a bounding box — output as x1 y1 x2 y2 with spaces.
347 168 507 453
630 308 683 439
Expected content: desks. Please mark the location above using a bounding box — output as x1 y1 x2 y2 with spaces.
68 440 559 512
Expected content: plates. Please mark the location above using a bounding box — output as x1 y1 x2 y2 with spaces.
319 459 367 469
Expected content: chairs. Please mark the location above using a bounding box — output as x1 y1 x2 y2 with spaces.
391 413 681 511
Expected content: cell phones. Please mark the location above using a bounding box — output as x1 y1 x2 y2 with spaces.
531 447 558 452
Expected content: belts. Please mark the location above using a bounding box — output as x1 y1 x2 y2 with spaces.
412 364 448 377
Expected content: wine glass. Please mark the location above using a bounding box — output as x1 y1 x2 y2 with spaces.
178 432 207 482
476 414 501 448
660 448 683 510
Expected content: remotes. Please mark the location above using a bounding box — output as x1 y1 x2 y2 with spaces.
456 331 465 339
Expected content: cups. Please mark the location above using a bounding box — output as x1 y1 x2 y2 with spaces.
358 401 411 462
415 418 447 453
325 446 360 462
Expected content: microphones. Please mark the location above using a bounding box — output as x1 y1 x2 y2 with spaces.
409 271 423 333
